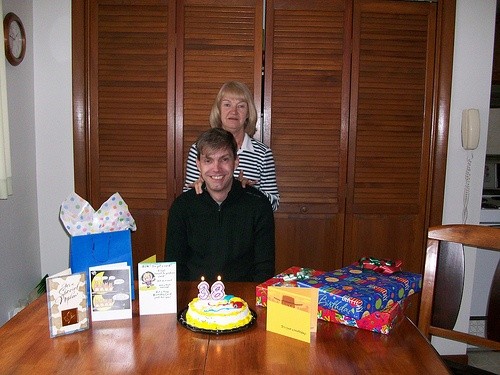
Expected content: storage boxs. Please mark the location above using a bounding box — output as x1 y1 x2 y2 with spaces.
297 257 424 320
254 265 414 335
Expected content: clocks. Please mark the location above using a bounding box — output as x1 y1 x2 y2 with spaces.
3 13 27 67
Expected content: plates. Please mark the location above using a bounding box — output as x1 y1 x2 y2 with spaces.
177 304 256 335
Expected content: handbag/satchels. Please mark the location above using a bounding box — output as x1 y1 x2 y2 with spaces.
68 230 137 308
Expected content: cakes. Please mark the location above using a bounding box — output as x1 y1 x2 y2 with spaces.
185 294 253 330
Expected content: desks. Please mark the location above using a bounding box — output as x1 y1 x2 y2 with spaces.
0 283 449 375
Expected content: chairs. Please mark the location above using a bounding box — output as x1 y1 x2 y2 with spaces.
417 224 500 375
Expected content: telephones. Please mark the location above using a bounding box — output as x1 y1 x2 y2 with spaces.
461 109 480 150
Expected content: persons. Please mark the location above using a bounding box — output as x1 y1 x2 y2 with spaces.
181 80 280 211
165 127 276 283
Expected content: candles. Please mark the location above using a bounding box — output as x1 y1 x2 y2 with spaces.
197 276 208 300
210 275 226 299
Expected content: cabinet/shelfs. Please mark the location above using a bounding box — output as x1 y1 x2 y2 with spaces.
72 0 457 323
